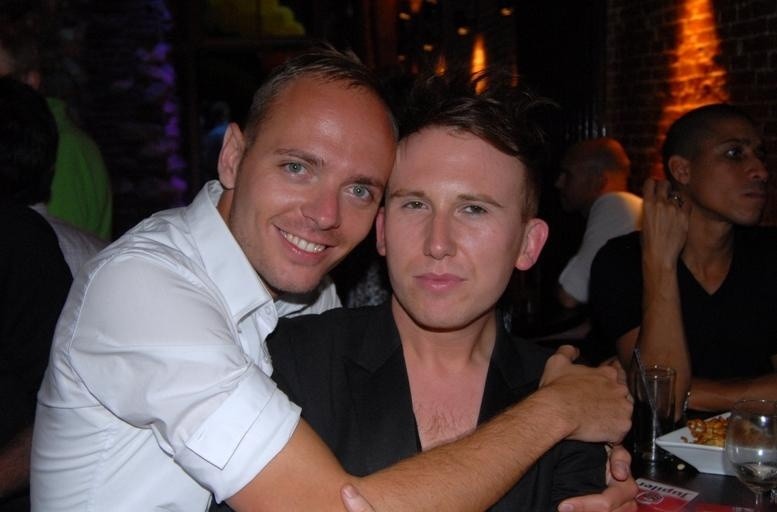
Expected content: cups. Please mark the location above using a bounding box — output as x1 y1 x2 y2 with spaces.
634 366 692 428
630 362 678 465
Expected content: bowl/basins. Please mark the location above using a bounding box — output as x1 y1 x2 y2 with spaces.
654 410 776 480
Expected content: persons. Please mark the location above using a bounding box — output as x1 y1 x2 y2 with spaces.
206 27 390 309
555 103 774 423
3 28 112 512
31 48 638 512
208 95 608 512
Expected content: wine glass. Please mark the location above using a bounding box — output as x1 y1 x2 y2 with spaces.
723 398 777 512
